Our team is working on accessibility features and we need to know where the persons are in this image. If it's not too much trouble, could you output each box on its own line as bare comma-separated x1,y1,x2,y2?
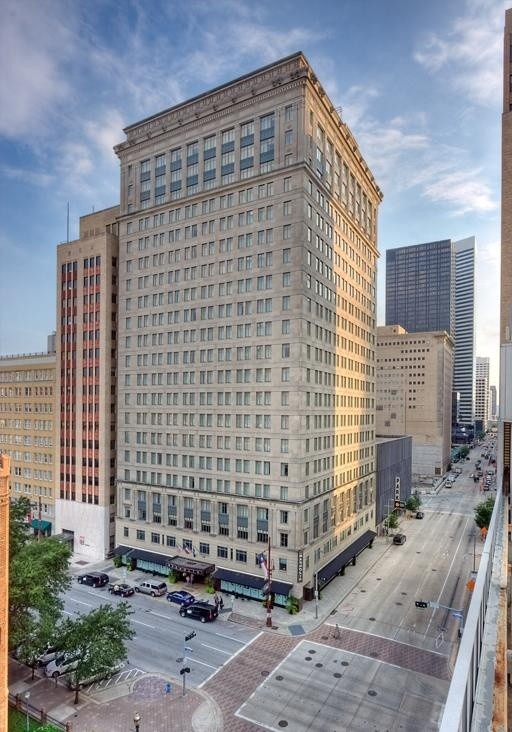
213,592,219,609
181,571,187,582
218,594,224,610
185,575,190,586
114,583,120,595
190,573,195,583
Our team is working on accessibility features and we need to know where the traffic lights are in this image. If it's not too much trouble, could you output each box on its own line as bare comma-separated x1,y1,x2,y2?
186,631,197,641
414,601,427,609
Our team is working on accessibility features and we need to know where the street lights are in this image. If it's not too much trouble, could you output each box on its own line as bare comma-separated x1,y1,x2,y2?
133,711,141,732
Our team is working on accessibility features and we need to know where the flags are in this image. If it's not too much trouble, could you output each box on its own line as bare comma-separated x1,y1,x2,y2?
192,546,199,558
176,542,183,554
257,552,269,582
183,543,191,555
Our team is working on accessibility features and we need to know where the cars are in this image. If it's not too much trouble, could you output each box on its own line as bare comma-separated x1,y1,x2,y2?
67,661,120,690
166,590,196,607
26,641,65,668
12,633,41,660
416,512,424,519
468,432,498,491
44,651,88,678
108,584,134,598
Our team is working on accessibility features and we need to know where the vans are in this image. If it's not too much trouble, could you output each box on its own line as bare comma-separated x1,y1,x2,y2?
393,533,406,545
179,601,218,623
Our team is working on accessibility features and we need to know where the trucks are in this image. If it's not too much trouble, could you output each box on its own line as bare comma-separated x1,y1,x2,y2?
445,467,462,488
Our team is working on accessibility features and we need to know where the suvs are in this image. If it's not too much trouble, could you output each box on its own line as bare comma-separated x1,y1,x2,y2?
135,579,167,597
77,571,110,588
